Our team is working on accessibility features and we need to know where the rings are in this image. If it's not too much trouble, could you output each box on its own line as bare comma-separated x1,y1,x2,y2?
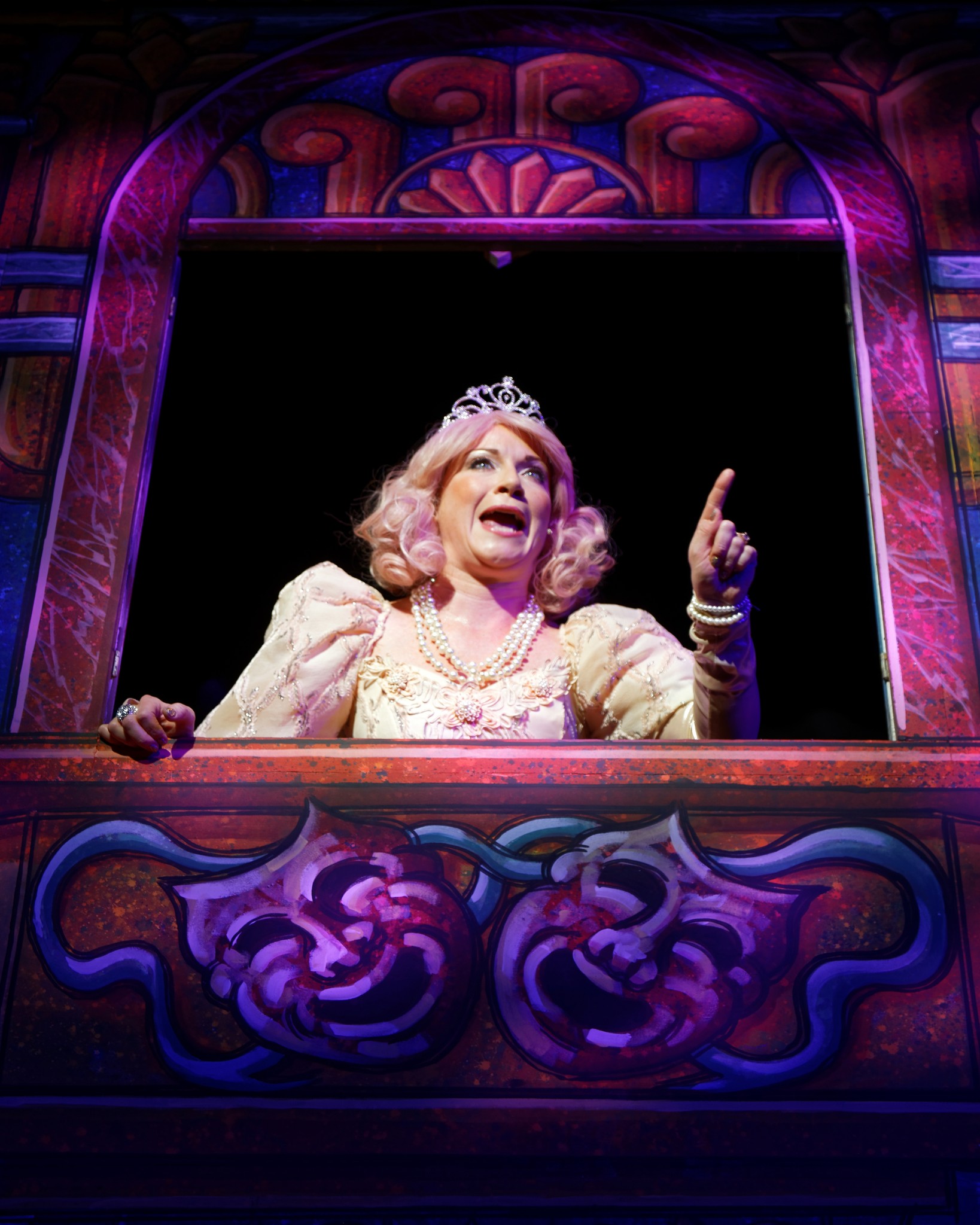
116,703,138,723
735,531,750,543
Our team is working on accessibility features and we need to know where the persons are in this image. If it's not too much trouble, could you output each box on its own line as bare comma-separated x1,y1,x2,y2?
95,373,760,759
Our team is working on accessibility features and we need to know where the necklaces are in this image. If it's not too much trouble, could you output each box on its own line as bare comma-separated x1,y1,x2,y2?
411,576,544,723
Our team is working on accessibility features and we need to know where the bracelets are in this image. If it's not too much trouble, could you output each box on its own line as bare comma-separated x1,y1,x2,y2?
684,591,752,643
